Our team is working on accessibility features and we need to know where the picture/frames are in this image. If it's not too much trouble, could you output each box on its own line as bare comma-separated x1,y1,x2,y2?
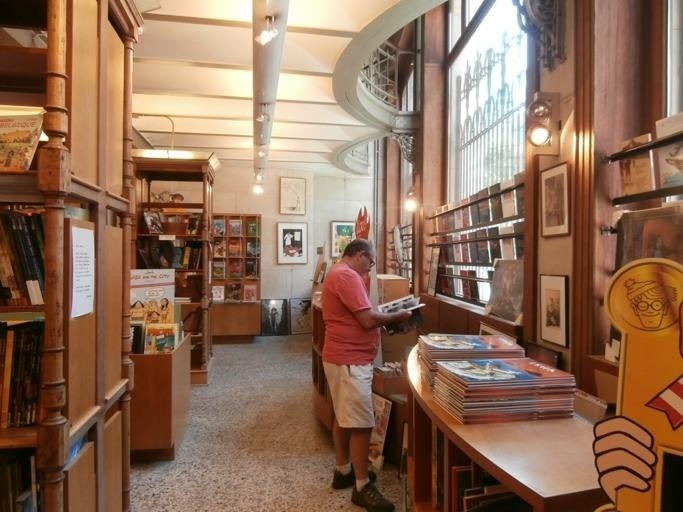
478,321,519,344
524,339,562,370
260,176,357,336
540,274,569,348
427,246,442,297
540,160,570,238
609,205,683,361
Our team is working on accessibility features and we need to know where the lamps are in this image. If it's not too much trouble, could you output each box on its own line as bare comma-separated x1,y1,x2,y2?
525,91,560,155
403,186,419,212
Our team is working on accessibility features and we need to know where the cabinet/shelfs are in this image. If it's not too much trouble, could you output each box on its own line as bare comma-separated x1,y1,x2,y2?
128,332,191,461
0,1,145,511
419,297,512,335
162,212,261,344
131,148,221,387
311,291,417,445
407,342,611,512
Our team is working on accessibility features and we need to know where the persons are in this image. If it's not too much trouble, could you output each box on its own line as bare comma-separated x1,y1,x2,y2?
623,280,676,329
284,231,295,255
592,414,657,506
319,236,397,511
269,304,279,336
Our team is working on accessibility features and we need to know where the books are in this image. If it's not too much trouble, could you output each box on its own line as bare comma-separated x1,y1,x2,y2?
0,102,44,507
211,219,258,302
129,208,202,355
615,132,656,197
451,464,532,511
655,111,683,188
377,295,426,335
415,333,576,424
425,170,525,302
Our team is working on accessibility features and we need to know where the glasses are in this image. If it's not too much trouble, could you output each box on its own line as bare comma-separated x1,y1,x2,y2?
368,258,375,268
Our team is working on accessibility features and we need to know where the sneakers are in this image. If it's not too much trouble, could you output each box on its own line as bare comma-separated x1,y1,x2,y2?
332,463,376,489
351,479,395,512
